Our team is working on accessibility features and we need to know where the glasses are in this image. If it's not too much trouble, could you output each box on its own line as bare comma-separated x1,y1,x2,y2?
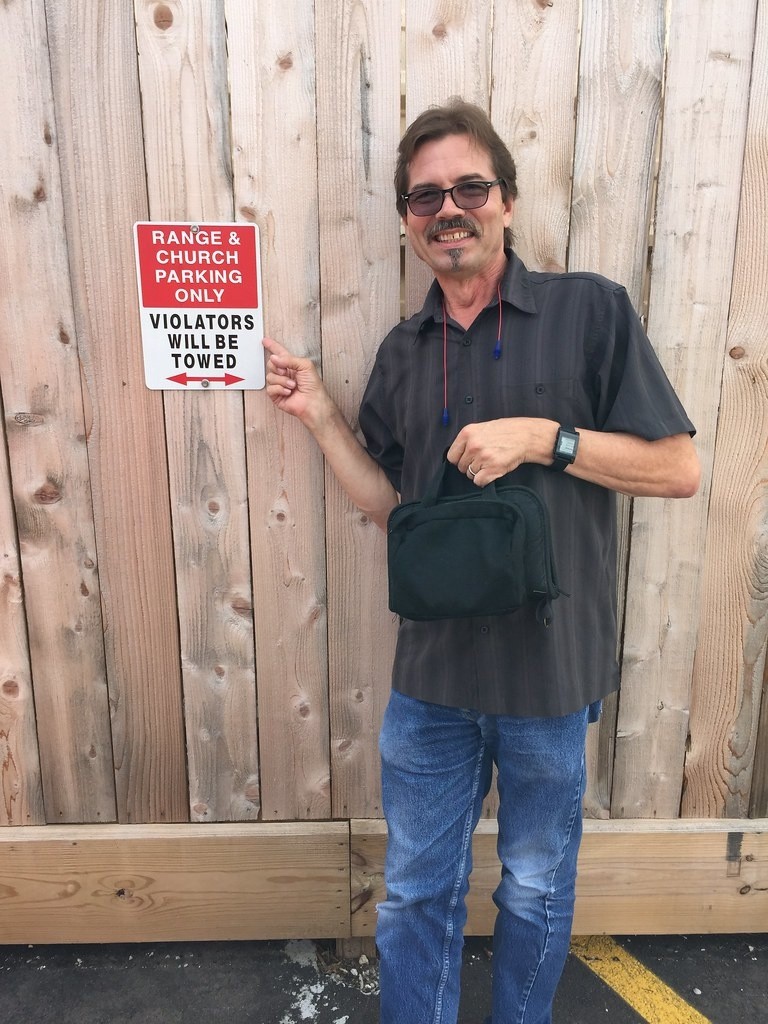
400,176,503,217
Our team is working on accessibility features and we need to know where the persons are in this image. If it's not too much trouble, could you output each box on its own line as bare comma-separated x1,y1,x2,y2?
256,92,703,1021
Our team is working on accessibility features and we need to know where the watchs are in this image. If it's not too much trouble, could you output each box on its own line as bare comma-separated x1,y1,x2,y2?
545,420,581,473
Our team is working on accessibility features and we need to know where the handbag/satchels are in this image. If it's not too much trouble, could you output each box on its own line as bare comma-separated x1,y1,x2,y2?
386,445,571,628
